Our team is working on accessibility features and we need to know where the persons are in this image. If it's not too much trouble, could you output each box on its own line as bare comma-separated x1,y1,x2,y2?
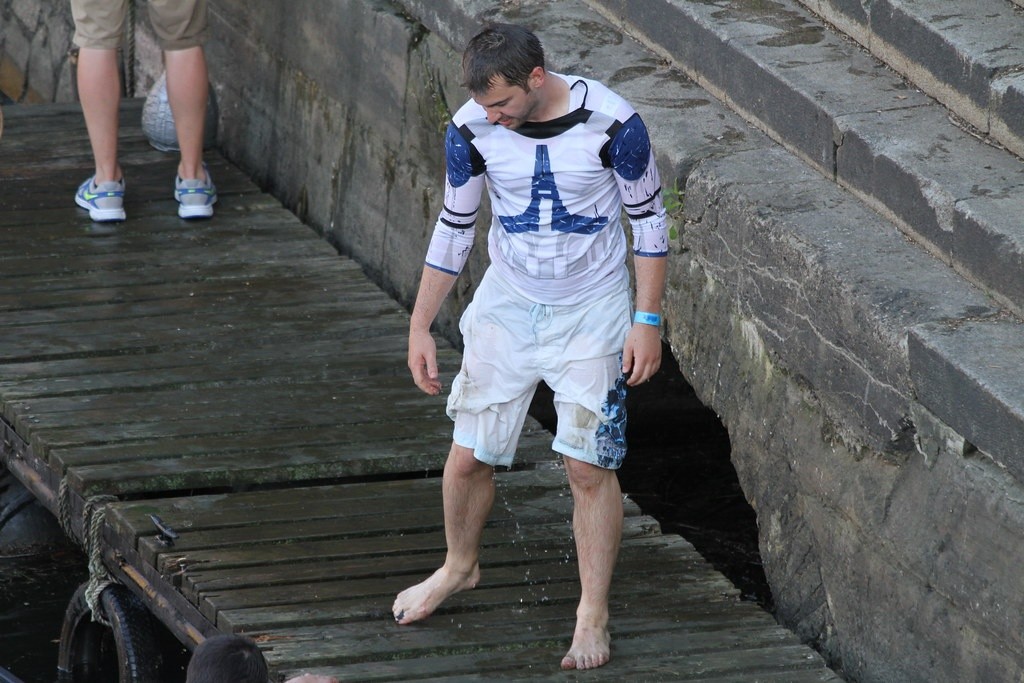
186,634,337,683
390,24,668,669
74,1,223,222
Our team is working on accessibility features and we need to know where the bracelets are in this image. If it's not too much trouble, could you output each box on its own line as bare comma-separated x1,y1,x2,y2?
634,311,660,327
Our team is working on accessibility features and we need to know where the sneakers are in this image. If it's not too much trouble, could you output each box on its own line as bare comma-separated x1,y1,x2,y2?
74,172,127,222
174,164,217,218
